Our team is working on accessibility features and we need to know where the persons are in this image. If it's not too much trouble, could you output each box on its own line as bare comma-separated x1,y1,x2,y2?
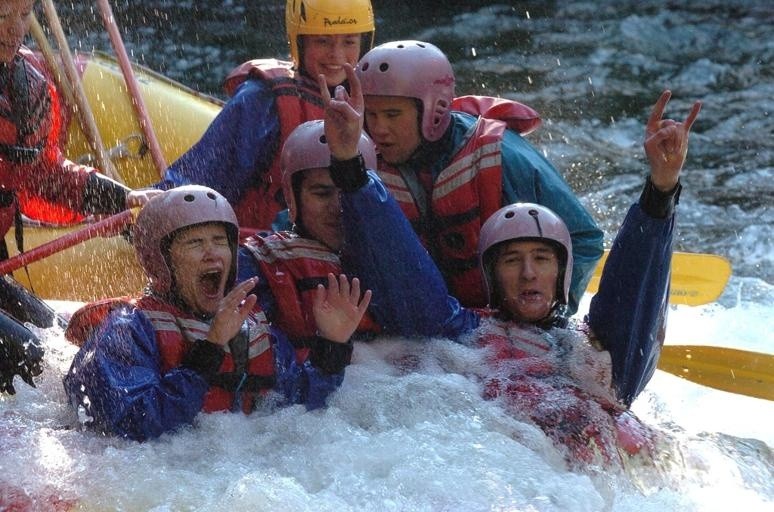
63,185,372,443
320,64,702,469
1,0,166,396
234,120,377,360
156,0,372,207
355,40,604,314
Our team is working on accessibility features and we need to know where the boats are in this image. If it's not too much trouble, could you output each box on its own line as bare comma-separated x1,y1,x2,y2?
0,49,773,306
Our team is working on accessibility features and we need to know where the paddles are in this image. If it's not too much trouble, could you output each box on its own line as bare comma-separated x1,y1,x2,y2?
656,344,773,402
586,248,730,306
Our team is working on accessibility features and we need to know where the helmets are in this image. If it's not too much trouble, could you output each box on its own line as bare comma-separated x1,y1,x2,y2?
475,201,572,311
285,0,375,68
279,118,378,231
349,39,455,146
129,183,240,299
